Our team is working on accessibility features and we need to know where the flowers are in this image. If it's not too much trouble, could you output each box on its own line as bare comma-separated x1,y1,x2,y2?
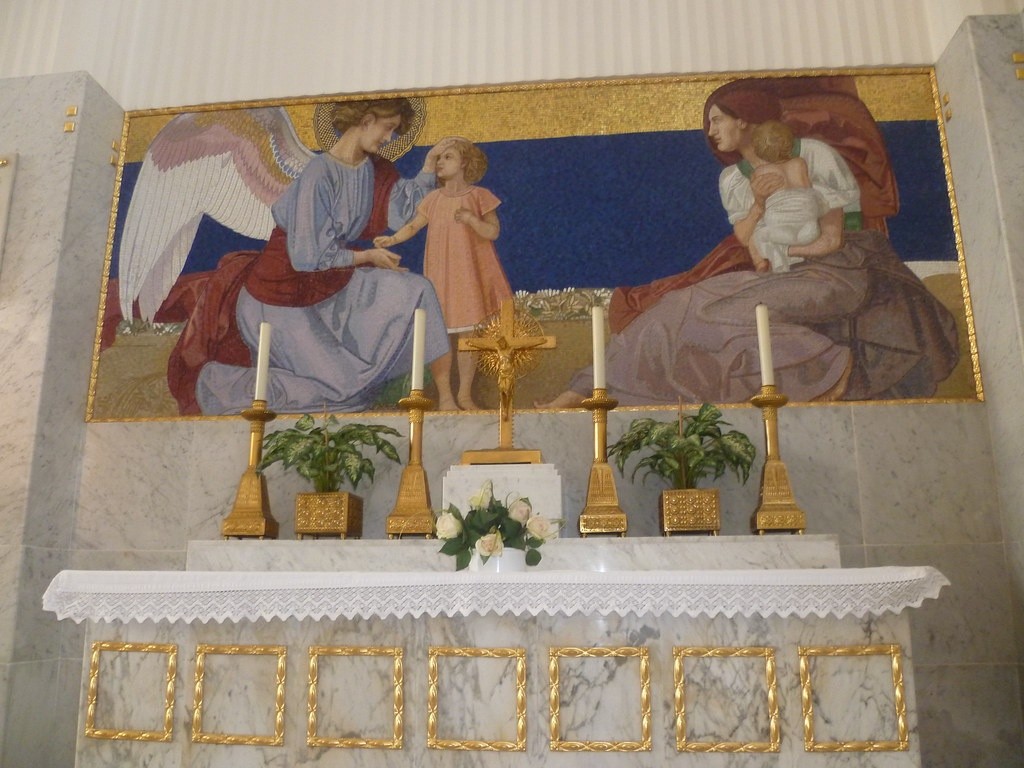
436,481,560,573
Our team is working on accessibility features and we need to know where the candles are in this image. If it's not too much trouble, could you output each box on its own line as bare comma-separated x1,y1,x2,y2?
755,302,774,390
407,305,428,389
590,305,606,393
254,322,273,403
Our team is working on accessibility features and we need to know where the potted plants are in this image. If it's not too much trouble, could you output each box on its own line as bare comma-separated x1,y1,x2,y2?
259,402,405,540
606,396,756,534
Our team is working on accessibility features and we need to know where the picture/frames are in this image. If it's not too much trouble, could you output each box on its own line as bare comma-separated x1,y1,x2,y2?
82,64,985,422
